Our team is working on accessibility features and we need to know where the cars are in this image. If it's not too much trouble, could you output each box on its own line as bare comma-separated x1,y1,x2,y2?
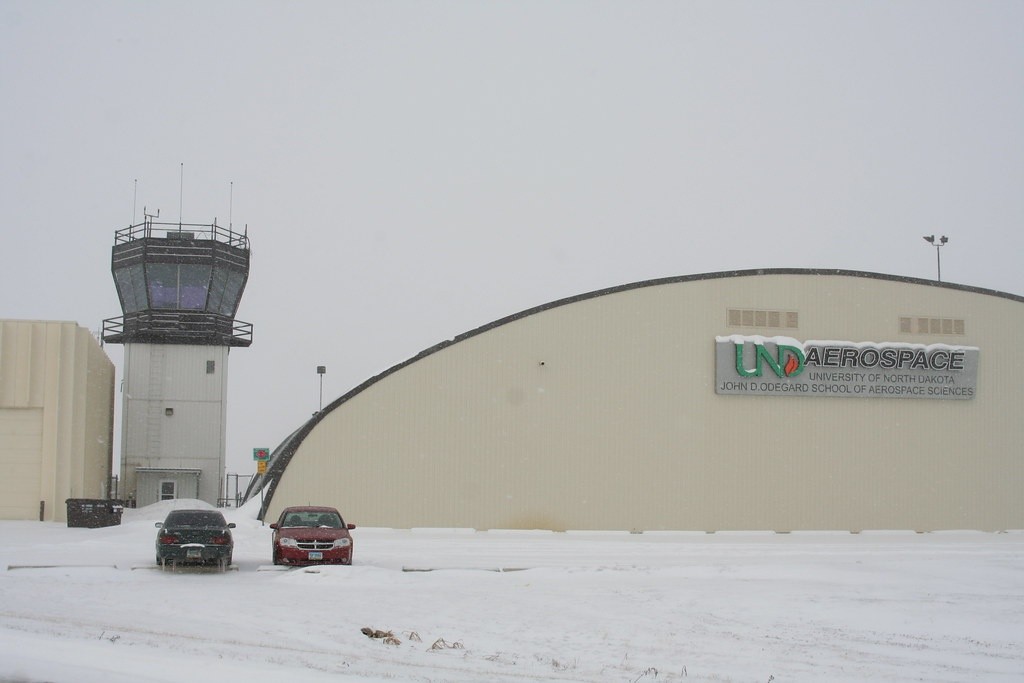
270,506,355,567
154,510,236,565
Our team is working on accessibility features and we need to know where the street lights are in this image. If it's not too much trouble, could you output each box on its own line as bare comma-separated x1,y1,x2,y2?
923,235,948,281
317,366,326,410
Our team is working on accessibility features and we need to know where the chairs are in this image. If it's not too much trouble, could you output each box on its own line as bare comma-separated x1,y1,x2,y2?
318,515,332,525
290,515,302,526
